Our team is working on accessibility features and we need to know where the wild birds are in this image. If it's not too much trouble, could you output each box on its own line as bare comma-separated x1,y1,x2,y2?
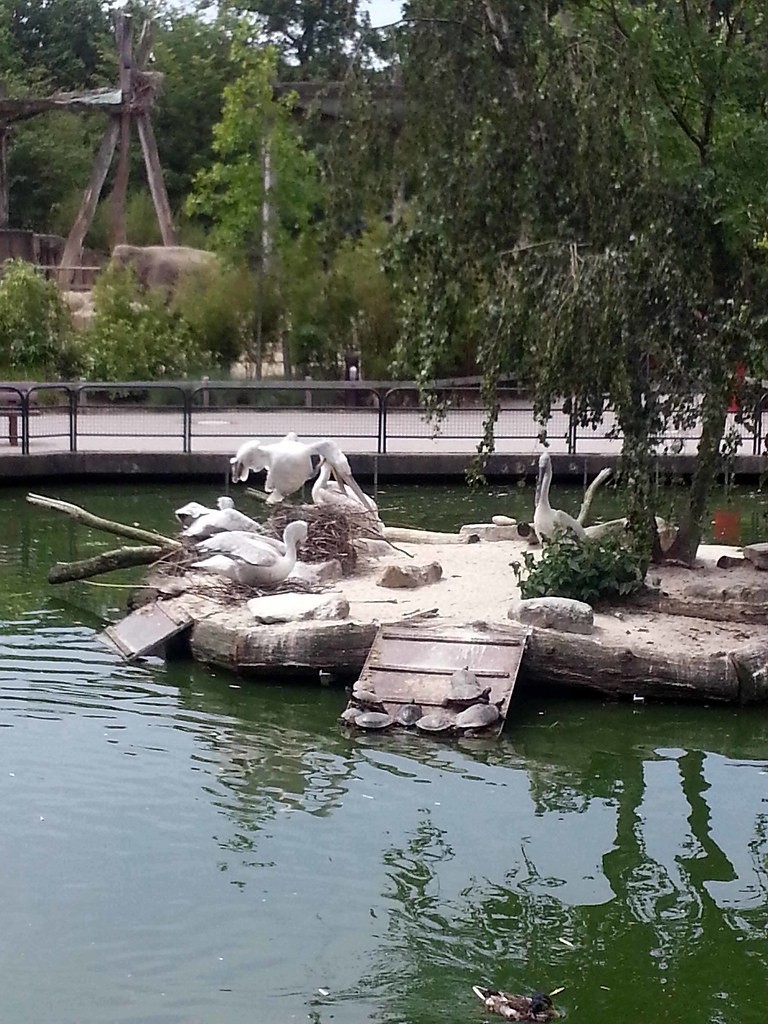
530,451,612,545
229,431,379,521
174,496,268,537
190,520,309,595
